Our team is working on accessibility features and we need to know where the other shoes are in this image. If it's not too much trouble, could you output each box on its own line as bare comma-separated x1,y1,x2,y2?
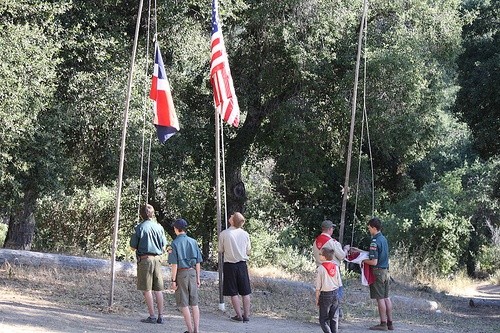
388,324,394,330
230,314,243,322
156,316,164,323
370,324,388,330
242,314,249,323
141,316,157,323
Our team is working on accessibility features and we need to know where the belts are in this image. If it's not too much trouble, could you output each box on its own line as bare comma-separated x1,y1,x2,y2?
177,267,194,271
139,254,159,259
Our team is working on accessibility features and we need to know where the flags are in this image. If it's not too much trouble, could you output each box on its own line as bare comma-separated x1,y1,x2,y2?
343,247,374,286
209,0,240,128
149,42,181,142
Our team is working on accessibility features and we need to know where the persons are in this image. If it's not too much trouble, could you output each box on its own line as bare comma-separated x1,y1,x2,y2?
129,203,167,324
314,247,344,333
312,219,351,318
217,212,252,322
360,219,394,330
167,218,203,333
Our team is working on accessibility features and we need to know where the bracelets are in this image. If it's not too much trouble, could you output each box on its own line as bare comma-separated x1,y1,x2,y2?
171,279,176,282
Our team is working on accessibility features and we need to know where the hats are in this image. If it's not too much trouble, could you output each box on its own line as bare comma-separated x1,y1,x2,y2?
321,221,336,229
170,218,188,230
